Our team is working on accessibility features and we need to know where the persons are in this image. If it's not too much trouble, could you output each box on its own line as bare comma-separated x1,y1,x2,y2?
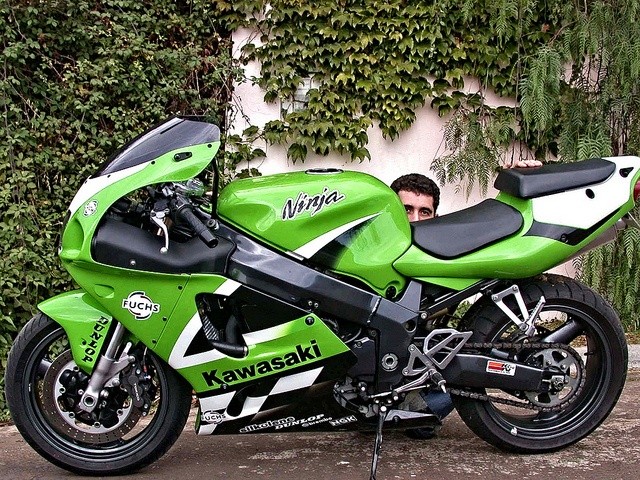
356,173,454,439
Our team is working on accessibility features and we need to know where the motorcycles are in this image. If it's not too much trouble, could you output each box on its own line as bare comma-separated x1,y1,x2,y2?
4,117,640,476
